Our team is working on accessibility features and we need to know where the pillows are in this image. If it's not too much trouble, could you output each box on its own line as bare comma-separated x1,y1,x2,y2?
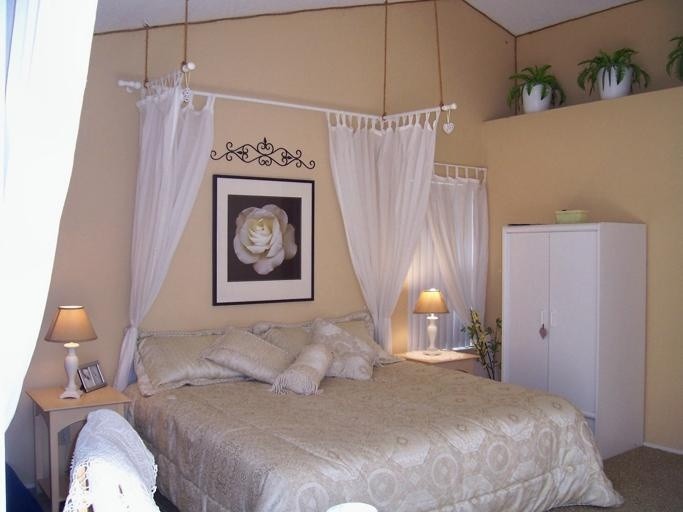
137,313,405,397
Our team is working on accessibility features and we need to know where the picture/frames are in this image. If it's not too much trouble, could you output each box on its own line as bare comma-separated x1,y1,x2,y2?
78,363,107,394
212,174,315,306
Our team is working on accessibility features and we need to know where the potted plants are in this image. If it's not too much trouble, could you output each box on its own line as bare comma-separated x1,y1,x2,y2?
504,47,652,115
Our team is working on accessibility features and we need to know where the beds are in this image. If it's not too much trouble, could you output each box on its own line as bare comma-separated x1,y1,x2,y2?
130,309,581,511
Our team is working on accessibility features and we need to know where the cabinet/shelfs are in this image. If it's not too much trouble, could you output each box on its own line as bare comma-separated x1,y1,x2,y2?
502,227,647,460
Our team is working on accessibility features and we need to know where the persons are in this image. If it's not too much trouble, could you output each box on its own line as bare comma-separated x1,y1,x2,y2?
82,368,100,389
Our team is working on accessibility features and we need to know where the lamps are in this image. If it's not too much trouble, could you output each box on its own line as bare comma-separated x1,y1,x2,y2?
412,288,451,356
44,305,98,399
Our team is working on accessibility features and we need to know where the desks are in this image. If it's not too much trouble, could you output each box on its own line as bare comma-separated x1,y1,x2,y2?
26,383,132,512
406,350,479,375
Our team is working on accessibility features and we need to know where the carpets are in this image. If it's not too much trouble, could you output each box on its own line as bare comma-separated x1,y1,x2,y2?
550,444,681,511
